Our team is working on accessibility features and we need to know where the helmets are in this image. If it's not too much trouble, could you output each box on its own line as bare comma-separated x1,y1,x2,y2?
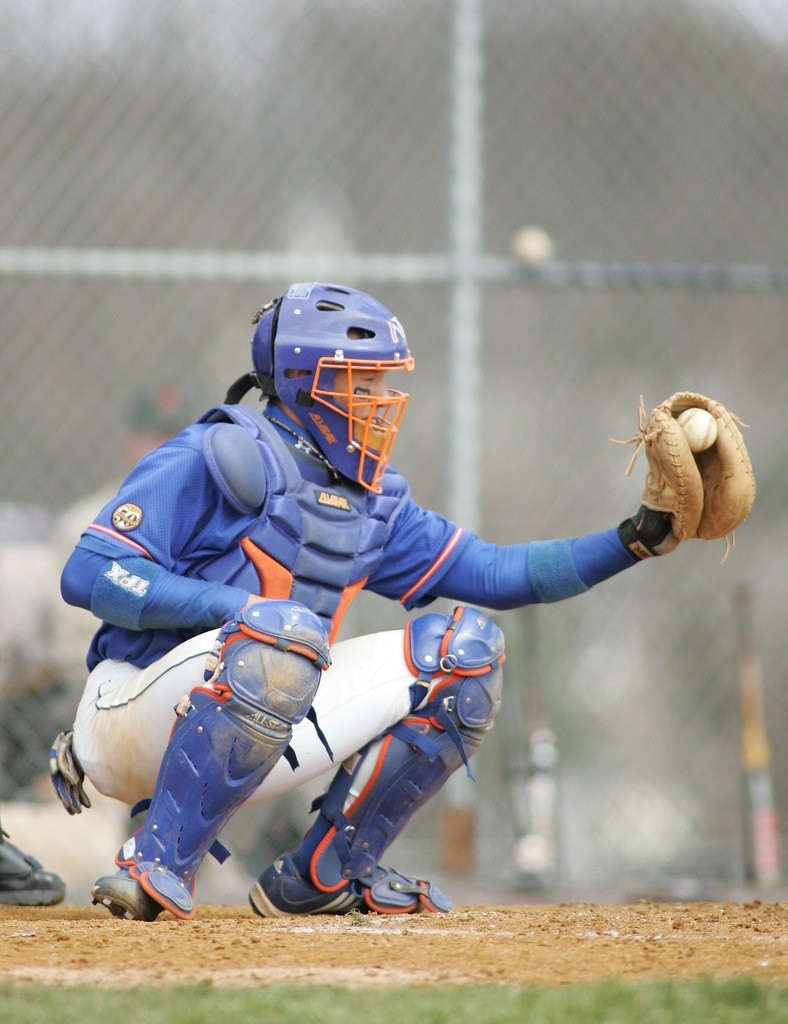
250,282,415,495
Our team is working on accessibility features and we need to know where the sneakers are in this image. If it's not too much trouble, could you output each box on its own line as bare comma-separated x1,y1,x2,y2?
248,846,424,917
90,866,160,922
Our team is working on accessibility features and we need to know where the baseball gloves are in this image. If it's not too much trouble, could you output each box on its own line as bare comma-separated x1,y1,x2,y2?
629,391,758,566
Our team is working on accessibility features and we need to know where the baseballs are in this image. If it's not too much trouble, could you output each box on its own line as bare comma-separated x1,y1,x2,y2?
674,406,721,453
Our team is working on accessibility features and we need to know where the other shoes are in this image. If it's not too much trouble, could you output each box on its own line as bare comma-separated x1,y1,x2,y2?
0,828,66,906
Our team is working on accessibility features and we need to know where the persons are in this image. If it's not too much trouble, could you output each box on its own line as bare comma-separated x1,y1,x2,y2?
56,286,757,925
